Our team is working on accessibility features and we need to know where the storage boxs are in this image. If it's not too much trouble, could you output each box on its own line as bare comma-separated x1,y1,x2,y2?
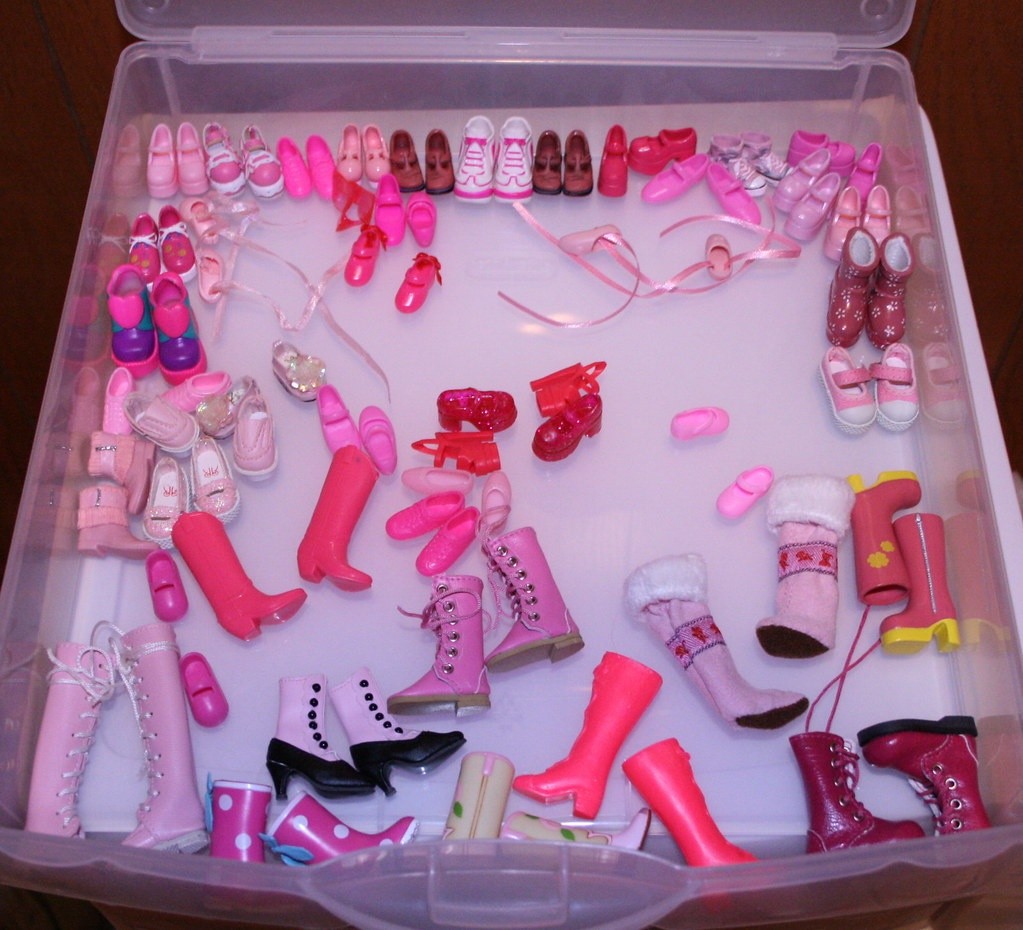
0,0,1023,930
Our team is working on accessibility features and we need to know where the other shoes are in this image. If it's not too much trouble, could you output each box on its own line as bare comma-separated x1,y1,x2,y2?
44,115,994,871
